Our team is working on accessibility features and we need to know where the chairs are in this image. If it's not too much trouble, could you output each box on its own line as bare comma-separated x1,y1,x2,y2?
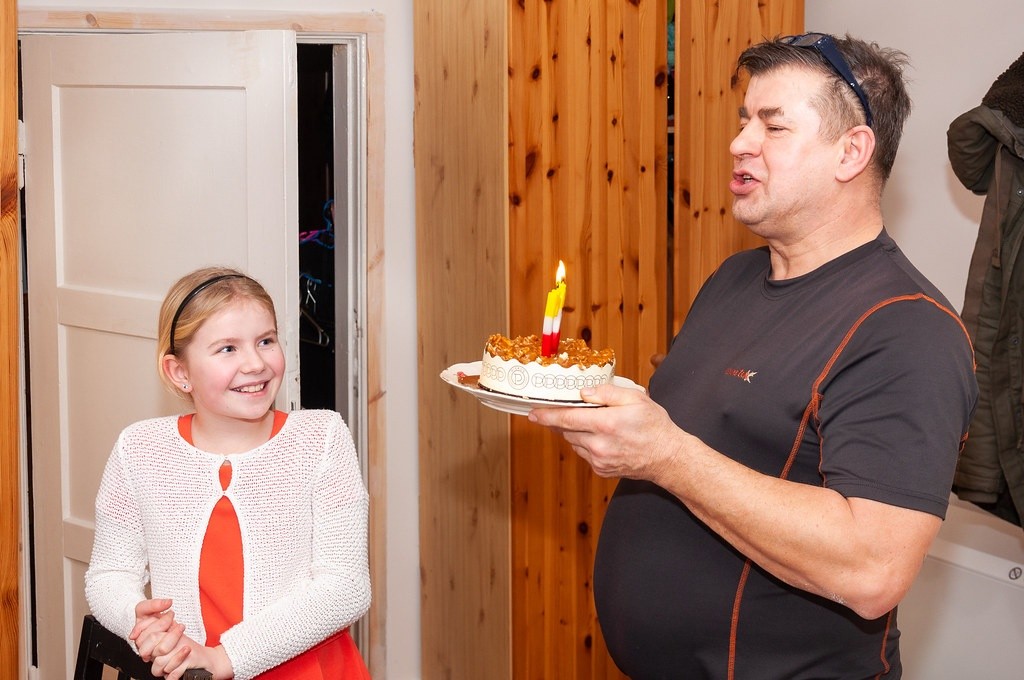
73,615,213,680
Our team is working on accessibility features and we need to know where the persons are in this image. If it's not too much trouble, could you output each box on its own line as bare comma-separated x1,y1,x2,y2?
85,266,375,680
526,38,976,680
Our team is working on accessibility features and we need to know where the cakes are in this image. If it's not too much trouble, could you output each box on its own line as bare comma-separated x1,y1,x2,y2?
477,333,617,400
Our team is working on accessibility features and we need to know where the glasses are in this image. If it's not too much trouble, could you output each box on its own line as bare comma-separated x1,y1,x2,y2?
775,35,872,125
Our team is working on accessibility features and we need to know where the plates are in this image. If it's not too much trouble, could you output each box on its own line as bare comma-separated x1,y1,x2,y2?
439,361,646,417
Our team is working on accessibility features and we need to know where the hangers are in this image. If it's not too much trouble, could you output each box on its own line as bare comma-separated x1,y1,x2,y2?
298,198,334,347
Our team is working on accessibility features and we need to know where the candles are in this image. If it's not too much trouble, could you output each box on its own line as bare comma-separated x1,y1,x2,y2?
542,260,568,356
